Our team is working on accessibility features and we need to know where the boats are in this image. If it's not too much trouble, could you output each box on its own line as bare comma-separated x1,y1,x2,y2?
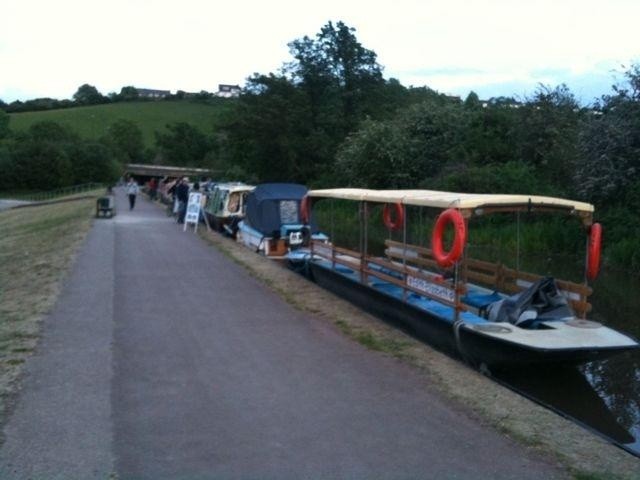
283,186,639,375
204,182,257,233
236,182,331,262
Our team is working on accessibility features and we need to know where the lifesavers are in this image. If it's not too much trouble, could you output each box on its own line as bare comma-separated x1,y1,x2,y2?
588,223,601,278
431,210,466,268
302,197,311,224
384,202,403,230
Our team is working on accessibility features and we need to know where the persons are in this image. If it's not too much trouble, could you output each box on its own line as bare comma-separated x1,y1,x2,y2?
126,177,139,210
149,176,217,224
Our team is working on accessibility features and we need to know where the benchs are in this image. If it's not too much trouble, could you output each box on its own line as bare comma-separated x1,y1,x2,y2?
309,238,594,330
